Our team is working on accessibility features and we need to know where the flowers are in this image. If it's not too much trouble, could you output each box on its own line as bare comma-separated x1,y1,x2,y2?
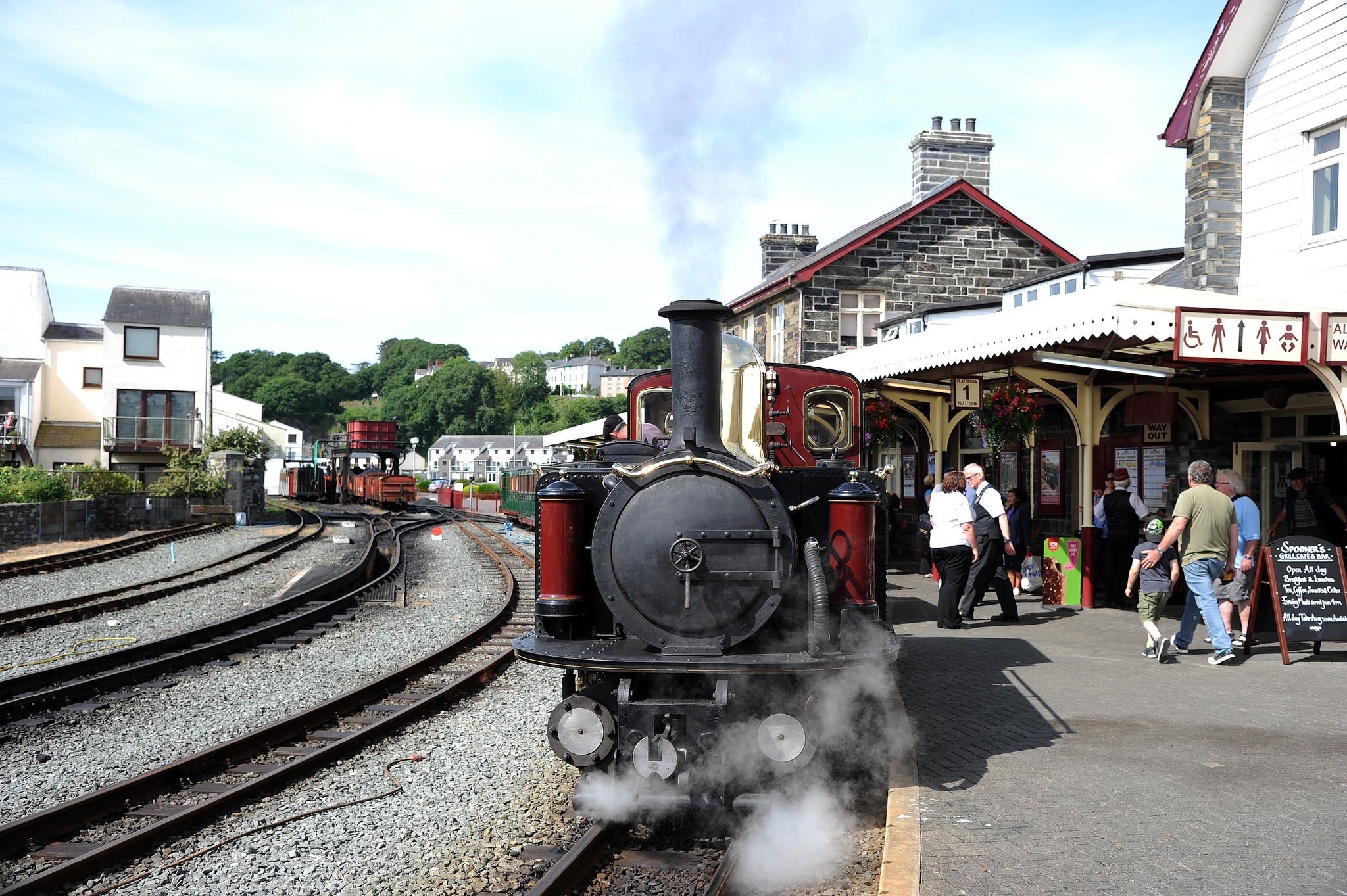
863,395,902,454
965,382,1044,449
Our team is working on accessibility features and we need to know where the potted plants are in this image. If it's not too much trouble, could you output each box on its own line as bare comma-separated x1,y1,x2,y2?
417,480,432,493
463,485,479,497
477,483,501,500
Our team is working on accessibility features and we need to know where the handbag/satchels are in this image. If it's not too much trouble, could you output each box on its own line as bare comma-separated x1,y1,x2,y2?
1021,550,1043,591
1092,526,1103,553
917,513,933,540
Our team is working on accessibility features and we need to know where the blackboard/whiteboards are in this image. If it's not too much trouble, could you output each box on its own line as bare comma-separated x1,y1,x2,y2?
1245,534,1347,640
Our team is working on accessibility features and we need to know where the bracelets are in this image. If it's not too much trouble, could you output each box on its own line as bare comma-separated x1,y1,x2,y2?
1097,496,1101,499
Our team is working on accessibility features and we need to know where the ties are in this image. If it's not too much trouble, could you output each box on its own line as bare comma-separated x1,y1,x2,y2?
1144,515,1165,543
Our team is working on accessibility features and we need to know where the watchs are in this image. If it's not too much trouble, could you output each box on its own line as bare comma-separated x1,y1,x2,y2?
1004,538,1012,543
1156,545,1167,554
1242,554,1253,560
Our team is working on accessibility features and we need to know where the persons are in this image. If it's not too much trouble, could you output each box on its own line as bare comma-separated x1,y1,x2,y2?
1092,468,1165,609
1125,515,1180,663
1002,489,1033,595
885,502,897,540
1266,467,1347,544
603,415,670,448
1204,469,1262,647
1140,459,1239,664
958,464,1020,624
929,471,972,629
0,411,18,443
920,467,1010,607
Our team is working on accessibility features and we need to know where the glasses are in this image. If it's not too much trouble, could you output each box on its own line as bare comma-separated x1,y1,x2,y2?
1105,476,1114,482
1215,481,1229,485
966,472,979,480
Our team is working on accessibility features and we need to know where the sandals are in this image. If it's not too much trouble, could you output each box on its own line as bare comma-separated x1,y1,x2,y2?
1232,634,1257,646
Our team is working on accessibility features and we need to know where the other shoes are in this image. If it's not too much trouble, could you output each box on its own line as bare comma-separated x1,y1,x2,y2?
1013,587,1022,596
991,614,1019,623
961,615,974,620
943,623,971,630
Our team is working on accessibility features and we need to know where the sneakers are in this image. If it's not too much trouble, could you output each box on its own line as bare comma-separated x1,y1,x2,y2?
1155,636,1170,663
1170,634,1189,654
1207,648,1235,665
1142,644,1157,658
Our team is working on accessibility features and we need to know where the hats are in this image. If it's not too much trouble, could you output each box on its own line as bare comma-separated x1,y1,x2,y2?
1114,468,1130,481
603,414,623,441
1287,467,1308,480
943,466,957,475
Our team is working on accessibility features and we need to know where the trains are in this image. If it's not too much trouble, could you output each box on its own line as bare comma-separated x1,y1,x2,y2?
278,467,325,501
497,299,894,831
322,472,416,510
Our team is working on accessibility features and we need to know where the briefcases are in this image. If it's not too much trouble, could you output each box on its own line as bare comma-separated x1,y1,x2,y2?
1204,633,1235,643
